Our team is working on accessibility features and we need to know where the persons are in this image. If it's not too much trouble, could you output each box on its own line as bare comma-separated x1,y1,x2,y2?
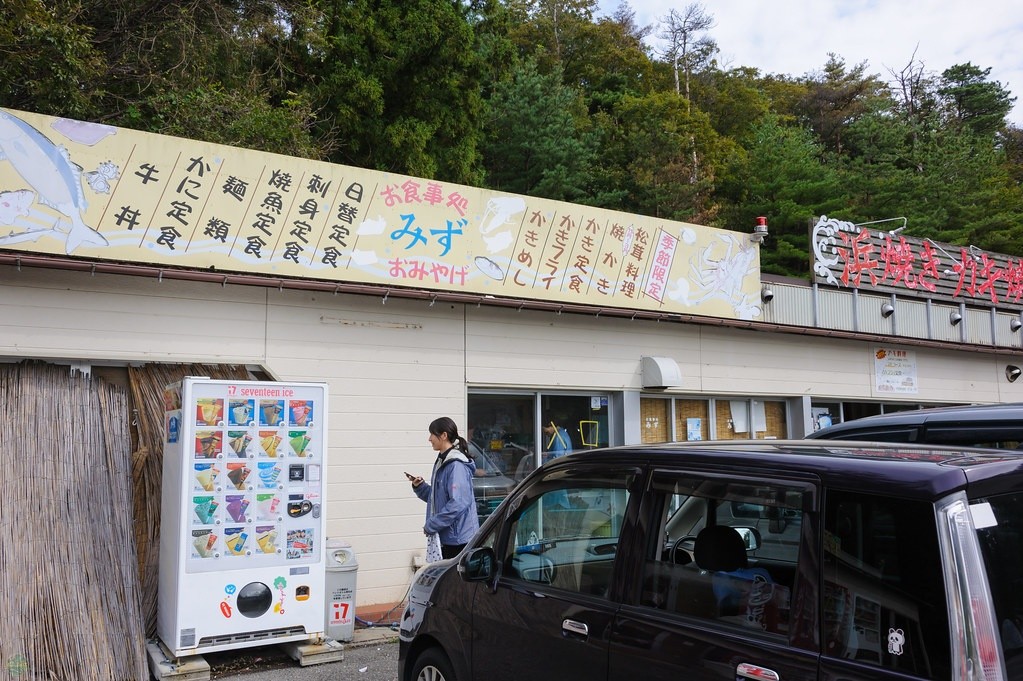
542,417,572,538
406,417,479,559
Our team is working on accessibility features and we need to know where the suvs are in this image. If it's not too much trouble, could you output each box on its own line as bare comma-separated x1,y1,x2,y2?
514,450,581,484
469,441,516,528
398,405,1023,681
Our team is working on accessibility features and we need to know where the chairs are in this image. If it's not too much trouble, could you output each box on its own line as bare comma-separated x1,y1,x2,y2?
694,526,778,631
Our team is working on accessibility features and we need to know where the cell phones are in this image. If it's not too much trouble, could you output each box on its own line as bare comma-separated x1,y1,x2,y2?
404,472,422,483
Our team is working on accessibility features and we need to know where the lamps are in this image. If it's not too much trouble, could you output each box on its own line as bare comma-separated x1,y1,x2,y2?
950,312,962,326
761,288,773,304
881,304,894,318
1011,319,1022,332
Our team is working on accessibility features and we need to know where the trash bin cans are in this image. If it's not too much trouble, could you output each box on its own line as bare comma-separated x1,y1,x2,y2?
590,520,612,537
325,542,359,644
